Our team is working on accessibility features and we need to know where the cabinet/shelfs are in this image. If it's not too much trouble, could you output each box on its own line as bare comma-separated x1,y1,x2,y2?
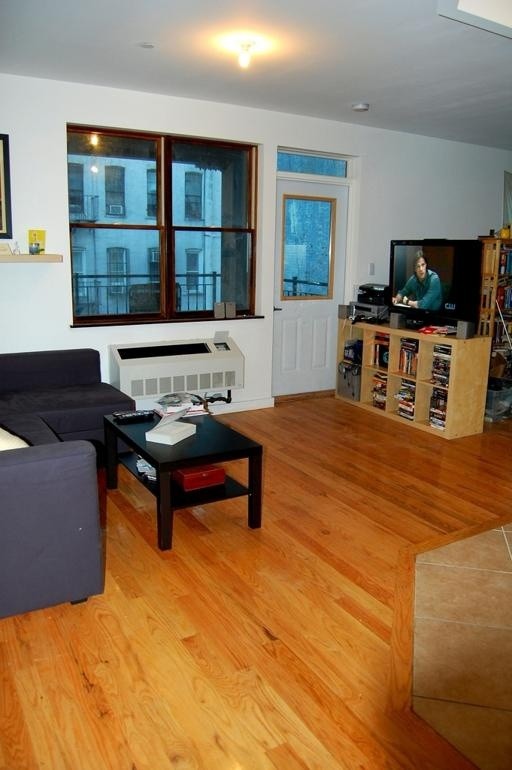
335,318,492,440
477,236,512,351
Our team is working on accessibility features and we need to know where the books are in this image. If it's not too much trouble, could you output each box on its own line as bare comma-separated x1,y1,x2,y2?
392,380,416,421
428,388,447,431
482,289,511,309
145,408,197,445
482,320,512,344
164,404,204,412
397,339,417,376
154,407,209,417
431,345,451,387
373,332,390,370
370,372,387,410
500,253,510,274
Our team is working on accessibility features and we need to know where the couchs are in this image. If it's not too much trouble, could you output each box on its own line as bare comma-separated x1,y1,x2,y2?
0,348,136,618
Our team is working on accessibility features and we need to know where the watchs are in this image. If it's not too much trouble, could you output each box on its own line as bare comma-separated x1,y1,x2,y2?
406,299,409,305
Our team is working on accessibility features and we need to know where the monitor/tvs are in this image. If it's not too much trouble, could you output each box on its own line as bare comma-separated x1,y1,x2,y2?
391,238,484,326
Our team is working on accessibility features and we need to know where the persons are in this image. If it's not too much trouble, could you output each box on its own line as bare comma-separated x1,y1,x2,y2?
392,249,443,311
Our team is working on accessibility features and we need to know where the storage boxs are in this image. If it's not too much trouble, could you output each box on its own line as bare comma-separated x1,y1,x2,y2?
173,467,225,492
485,384,512,422
337,371,360,401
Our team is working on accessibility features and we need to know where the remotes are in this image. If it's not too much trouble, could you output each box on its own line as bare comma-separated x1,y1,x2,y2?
118,410,155,419
112,410,137,417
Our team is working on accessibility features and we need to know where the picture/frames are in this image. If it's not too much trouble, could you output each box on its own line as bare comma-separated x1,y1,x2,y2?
1,133,13,239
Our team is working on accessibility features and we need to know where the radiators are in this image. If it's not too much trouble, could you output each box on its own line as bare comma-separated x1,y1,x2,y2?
108,332,245,400
